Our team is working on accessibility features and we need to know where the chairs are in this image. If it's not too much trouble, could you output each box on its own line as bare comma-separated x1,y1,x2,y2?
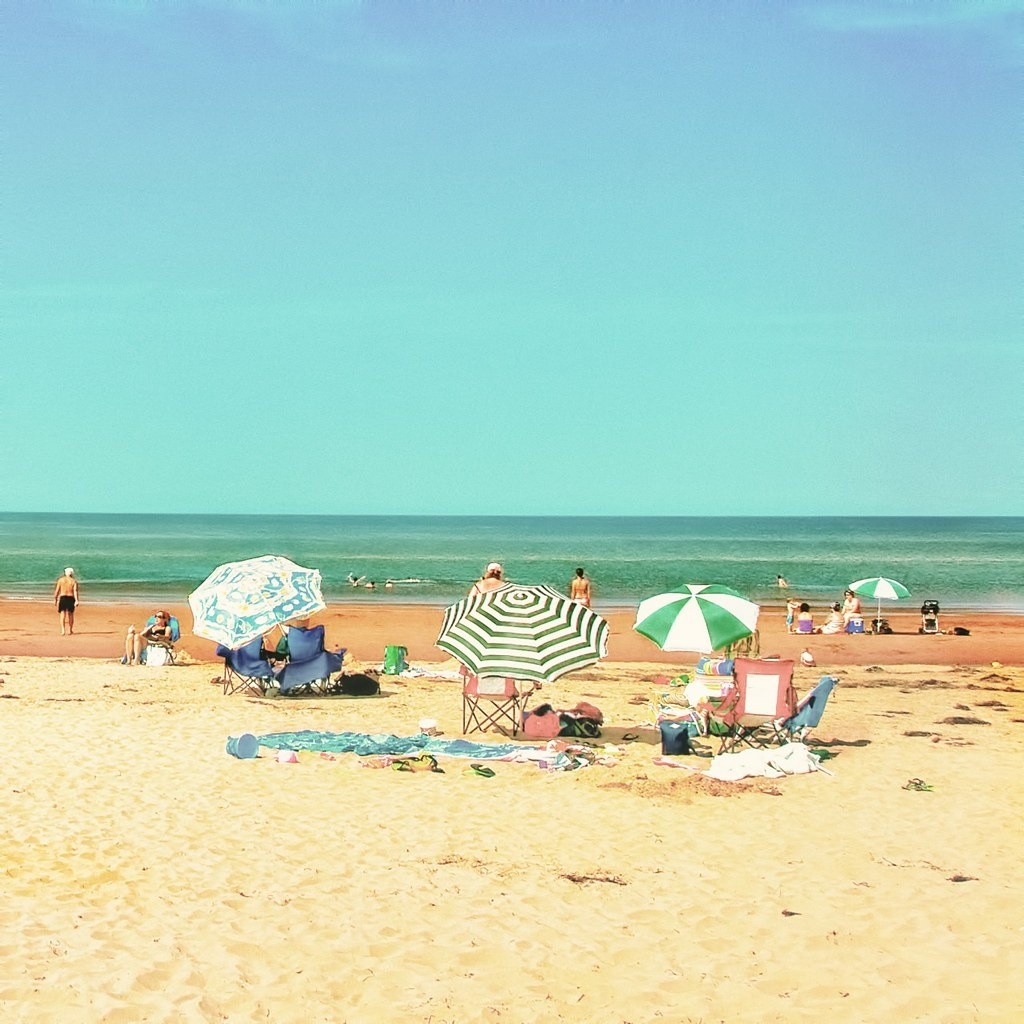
719,676,834,754
460,664,542,740
121,615,180,664
277,625,329,695
723,657,796,756
217,637,275,698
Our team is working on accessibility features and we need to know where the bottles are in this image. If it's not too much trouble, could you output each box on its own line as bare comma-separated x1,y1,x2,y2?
334,644,339,652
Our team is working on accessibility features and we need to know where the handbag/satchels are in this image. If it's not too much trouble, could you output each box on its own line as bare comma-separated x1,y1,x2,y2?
954,627,970,635
384,644,410,674
557,713,602,737
651,705,708,737
146,643,170,666
847,619,864,633
520,704,560,739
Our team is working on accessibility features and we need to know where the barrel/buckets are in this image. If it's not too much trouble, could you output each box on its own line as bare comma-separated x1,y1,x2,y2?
419,718,437,737
226,733,259,758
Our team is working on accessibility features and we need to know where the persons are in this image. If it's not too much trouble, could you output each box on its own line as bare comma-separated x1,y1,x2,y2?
364,581,376,587
466,562,507,599
346,574,367,587
777,574,786,590
54,567,81,638
841,588,863,628
274,618,310,661
568,568,592,609
124,610,173,665
384,580,392,588
796,603,815,635
784,597,801,633
813,602,847,634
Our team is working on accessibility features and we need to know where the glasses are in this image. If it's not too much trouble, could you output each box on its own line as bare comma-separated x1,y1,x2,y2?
155,614,164,619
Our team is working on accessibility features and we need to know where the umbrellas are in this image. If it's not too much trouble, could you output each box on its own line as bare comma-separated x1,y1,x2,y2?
632,583,761,659
847,576,912,635
434,581,612,734
186,553,328,652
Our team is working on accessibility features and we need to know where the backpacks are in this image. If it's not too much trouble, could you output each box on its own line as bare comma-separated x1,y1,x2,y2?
659,719,704,756
391,751,443,774
327,674,381,697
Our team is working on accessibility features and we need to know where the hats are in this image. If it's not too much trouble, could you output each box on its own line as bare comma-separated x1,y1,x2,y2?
488,563,503,575
